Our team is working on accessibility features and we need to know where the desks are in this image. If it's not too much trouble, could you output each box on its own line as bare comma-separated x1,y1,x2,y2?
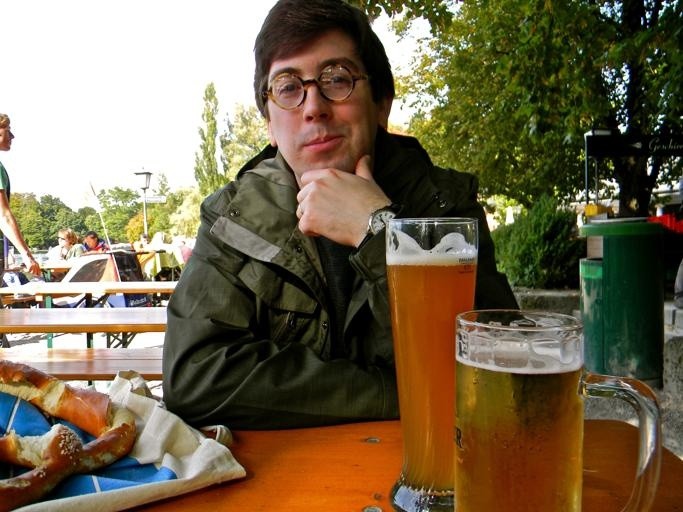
0,280,179,347
0,307,168,333
0,345,164,383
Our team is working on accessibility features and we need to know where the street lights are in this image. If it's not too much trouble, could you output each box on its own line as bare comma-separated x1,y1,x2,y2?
134,166,153,235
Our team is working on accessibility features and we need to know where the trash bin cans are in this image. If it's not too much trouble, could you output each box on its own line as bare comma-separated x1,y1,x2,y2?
576,218,666,394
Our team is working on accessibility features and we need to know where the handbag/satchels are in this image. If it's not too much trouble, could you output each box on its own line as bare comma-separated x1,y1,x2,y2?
1,370,248,512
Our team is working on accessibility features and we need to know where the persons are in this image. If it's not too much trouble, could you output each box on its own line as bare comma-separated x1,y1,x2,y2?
82,231,107,255
48,228,86,261
161,0,522,431
0,112,41,288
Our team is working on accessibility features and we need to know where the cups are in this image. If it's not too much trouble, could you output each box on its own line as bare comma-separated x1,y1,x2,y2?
455,308,663,508
386,217,478,507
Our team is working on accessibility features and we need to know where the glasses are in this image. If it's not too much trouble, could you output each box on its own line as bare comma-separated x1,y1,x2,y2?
57,237,66,241
262,64,370,110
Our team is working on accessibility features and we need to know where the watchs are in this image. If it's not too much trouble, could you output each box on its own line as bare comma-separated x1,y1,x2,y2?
356,202,403,248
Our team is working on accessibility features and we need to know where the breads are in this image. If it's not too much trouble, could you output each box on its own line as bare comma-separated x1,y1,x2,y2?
0,423,83,510
0,359,136,472
